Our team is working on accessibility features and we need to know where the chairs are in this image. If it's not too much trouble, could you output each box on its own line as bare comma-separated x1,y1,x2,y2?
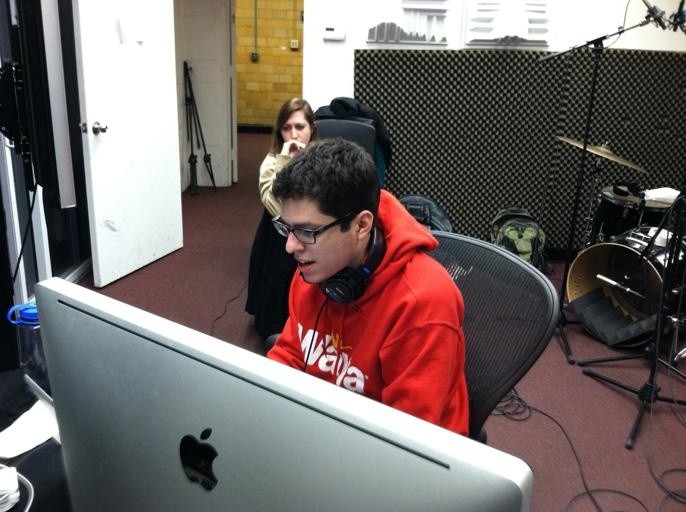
413,221,564,449
309,116,380,172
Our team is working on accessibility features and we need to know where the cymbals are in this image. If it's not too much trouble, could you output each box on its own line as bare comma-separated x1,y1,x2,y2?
556,135,649,175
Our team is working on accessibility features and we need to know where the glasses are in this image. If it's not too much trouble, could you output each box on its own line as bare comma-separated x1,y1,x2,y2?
272,213,359,245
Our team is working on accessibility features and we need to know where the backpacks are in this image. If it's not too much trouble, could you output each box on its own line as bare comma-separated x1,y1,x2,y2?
491,207,547,273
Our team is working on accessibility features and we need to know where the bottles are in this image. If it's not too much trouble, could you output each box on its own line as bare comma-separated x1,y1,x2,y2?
4,303,51,398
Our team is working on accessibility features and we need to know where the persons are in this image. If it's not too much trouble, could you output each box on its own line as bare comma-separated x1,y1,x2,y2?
266,137,469,436
243,98,317,337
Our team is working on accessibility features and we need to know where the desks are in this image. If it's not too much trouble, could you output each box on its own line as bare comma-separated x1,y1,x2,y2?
0,360,77,512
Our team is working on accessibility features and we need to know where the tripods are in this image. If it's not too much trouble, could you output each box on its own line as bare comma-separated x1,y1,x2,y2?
537,18,653,365
581,188,686,449
182,62,218,196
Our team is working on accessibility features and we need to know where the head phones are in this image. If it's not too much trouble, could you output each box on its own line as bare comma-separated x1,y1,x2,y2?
319,217,387,304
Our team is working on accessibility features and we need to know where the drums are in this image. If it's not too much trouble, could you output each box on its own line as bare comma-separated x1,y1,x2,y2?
565,187,686,348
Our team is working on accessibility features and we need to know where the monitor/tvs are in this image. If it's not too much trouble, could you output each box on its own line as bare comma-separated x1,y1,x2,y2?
34,276,535,512
